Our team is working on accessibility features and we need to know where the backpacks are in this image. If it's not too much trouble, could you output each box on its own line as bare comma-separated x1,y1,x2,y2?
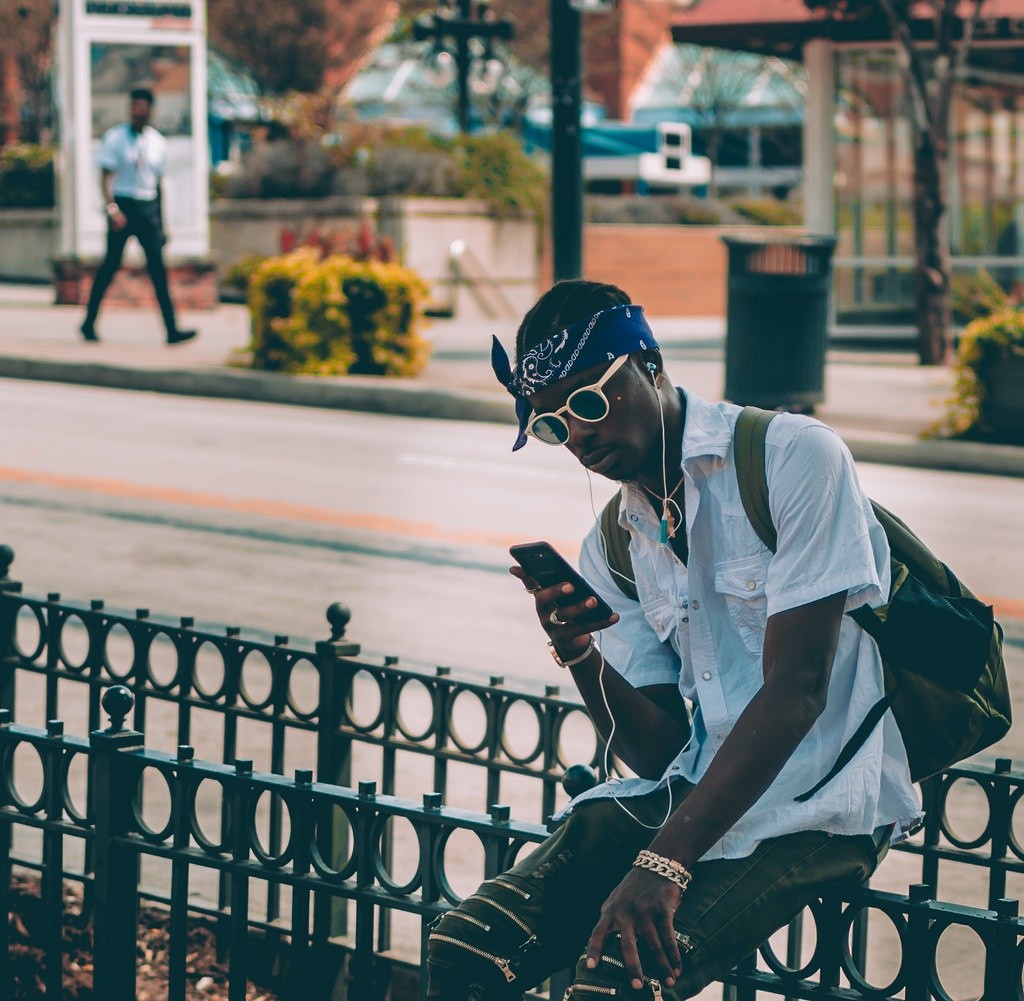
599,403,1012,783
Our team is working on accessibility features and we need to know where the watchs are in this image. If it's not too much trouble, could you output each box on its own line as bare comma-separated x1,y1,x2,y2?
547,636,596,668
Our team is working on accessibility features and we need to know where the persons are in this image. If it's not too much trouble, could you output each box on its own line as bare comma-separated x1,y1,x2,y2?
424,282,925,1001
80,88,197,345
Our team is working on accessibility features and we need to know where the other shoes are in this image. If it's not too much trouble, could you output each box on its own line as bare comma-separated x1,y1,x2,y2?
82,321,96,340
168,330,195,343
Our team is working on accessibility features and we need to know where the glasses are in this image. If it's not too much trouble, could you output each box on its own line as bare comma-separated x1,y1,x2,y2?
523,354,628,446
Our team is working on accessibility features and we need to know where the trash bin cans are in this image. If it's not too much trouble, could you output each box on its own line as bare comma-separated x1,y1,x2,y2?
719,233,837,417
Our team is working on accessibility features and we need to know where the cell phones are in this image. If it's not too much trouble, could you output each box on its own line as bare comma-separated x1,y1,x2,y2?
509,541,613,622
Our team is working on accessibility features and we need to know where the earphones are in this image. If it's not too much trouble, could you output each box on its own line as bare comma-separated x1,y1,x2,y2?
646,362,656,374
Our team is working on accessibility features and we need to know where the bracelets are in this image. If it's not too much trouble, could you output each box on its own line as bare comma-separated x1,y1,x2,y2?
633,850,692,892
